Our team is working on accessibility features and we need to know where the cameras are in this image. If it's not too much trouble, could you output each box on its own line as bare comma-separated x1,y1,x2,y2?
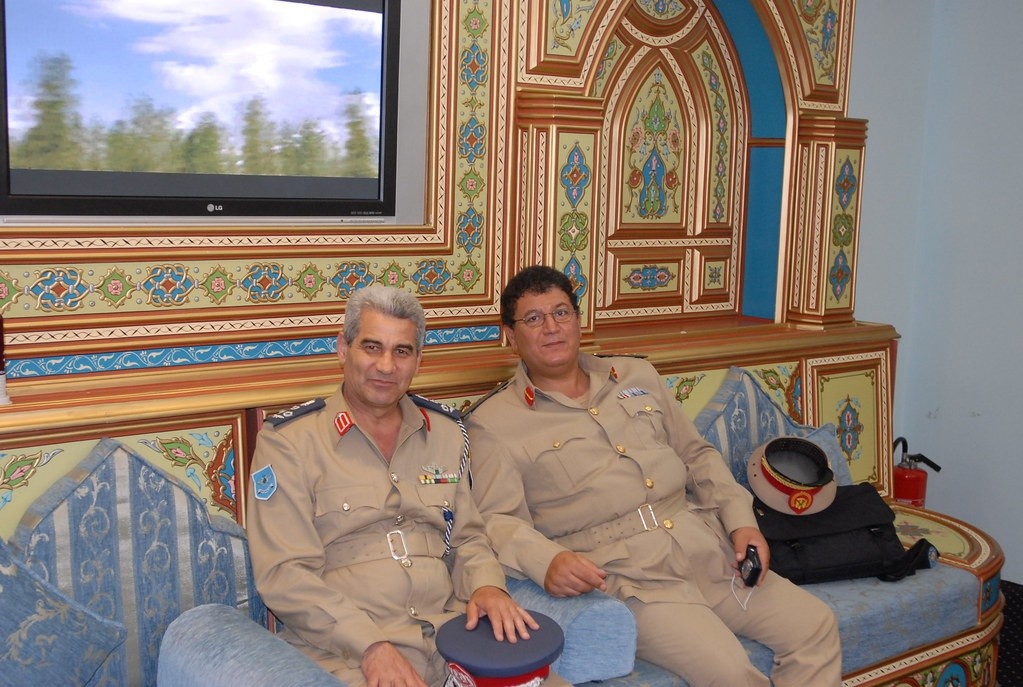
738,547,761,587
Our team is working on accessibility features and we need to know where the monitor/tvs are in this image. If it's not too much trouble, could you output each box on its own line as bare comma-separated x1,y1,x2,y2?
1,1,402,216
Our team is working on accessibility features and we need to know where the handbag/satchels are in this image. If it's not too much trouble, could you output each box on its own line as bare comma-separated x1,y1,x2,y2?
751,483,939,584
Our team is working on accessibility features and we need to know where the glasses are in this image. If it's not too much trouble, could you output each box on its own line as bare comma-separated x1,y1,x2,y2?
510,309,579,327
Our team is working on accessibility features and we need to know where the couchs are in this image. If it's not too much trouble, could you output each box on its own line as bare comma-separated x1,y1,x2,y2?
0,365,1008,687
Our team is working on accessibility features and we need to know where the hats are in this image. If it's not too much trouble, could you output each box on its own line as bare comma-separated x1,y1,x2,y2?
747,437,838,515
436,608,565,687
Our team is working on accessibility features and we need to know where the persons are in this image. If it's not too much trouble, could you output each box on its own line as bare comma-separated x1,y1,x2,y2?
457,266,845,686
249,284,579,687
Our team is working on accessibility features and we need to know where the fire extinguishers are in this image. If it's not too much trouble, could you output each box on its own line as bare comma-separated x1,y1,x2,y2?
892,436,941,509
